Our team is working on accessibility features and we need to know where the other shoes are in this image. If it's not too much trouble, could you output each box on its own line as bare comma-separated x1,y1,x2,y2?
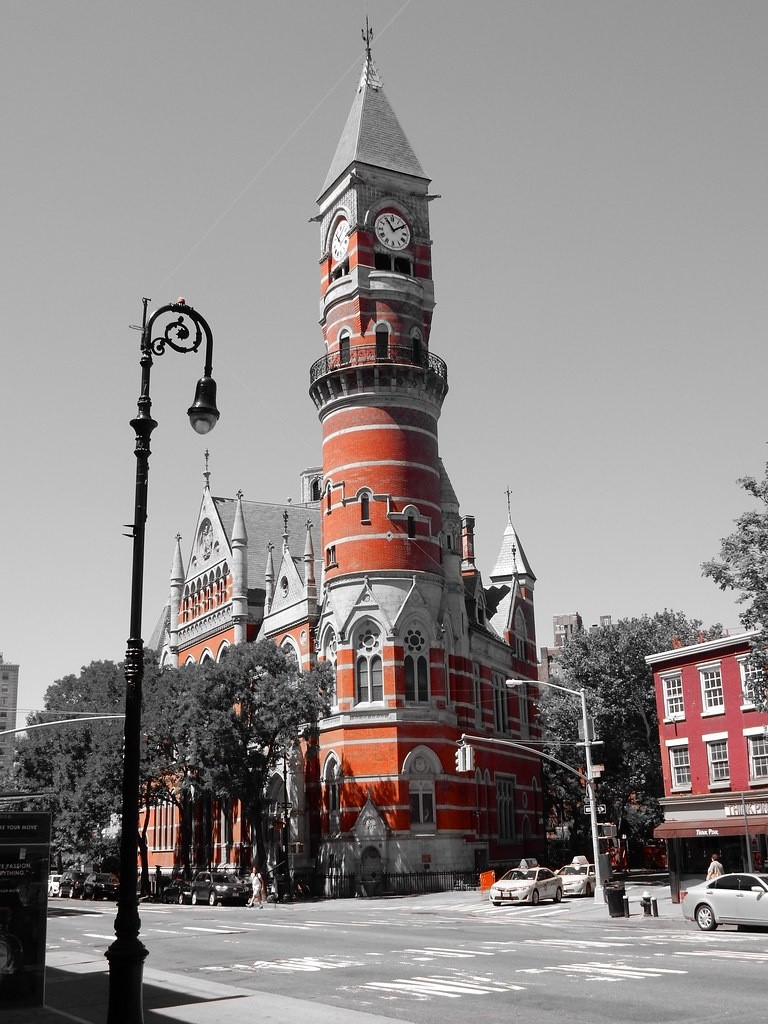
258,905,263,909
246,903,251,908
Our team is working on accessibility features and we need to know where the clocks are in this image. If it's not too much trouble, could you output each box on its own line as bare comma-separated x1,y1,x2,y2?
374,213,411,251
332,219,350,262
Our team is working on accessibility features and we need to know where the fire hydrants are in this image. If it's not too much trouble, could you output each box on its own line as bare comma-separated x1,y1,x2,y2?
641,890,652,918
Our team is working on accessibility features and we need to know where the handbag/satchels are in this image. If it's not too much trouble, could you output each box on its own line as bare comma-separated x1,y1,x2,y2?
710,862,722,879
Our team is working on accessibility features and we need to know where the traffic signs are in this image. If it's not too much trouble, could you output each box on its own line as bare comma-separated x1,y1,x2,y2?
276,802,292,808
584,804,606,815
582,795,599,804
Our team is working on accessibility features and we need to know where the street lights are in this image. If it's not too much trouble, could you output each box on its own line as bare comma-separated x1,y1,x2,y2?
105,296,219,1024
506,679,606,904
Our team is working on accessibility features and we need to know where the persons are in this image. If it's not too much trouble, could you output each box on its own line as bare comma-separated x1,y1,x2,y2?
706,854,724,881
245,866,263,908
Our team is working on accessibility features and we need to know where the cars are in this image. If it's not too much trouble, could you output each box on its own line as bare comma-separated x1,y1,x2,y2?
489,859,564,906
58,871,90,899
48,875,63,897
681,873,768,932
190,872,250,907
83,872,121,901
556,855,596,897
163,879,194,904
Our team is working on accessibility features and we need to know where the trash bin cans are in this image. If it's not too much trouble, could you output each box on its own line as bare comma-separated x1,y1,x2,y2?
276,875,292,899
606,880,625,917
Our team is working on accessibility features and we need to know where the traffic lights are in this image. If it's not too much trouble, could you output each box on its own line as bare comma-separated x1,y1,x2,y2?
466,746,476,771
601,825,617,837
455,749,466,772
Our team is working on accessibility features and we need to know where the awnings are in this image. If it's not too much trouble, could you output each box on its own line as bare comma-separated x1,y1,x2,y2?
654,817,768,839
